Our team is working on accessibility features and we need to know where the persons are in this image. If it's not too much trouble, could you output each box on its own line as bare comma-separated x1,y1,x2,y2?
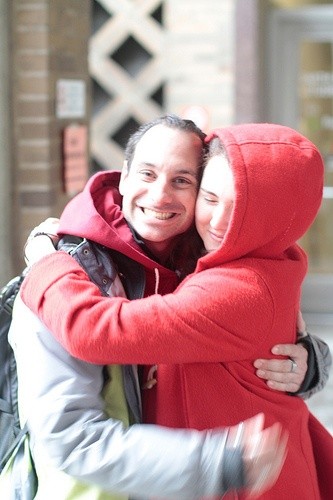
6,113,333,500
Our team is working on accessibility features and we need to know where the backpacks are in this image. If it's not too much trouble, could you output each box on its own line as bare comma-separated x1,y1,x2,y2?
0,233,95,500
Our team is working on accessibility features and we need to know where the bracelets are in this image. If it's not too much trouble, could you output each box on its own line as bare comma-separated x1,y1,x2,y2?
23,231,56,262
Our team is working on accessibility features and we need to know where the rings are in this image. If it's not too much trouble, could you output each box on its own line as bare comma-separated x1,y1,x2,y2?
288,358,295,373
